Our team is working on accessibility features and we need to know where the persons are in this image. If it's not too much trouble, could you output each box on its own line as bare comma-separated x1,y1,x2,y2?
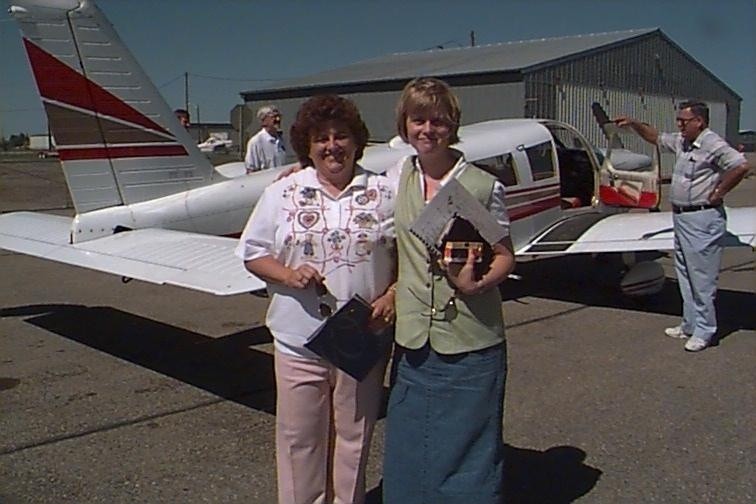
610,92,748,352
231,92,401,504
270,74,517,504
175,107,191,134
242,104,288,174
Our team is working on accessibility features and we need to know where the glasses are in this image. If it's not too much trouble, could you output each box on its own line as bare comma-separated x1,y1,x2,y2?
676,117,696,125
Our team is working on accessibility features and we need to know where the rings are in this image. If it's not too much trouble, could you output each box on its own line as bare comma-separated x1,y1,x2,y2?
384,317,390,323
389,321,394,325
299,276,304,282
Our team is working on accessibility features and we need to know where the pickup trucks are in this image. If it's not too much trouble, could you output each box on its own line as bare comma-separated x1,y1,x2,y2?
195,137,233,152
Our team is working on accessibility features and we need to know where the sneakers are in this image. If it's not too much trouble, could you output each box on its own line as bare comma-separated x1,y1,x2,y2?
664,325,690,340
684,336,711,352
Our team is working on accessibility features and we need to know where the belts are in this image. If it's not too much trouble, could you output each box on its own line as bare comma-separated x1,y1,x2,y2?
673,204,714,213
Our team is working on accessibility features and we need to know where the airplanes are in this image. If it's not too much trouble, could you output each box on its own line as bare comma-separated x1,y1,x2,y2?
0,0,756,294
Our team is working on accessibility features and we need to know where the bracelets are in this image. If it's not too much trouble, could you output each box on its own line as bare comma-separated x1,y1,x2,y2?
715,186,724,199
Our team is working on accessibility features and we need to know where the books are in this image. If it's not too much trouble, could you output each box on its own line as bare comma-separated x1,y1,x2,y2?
410,176,508,275
436,211,494,280
300,291,395,385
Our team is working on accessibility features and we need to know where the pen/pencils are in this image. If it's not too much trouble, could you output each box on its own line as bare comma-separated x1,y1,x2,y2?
355,292,374,310
